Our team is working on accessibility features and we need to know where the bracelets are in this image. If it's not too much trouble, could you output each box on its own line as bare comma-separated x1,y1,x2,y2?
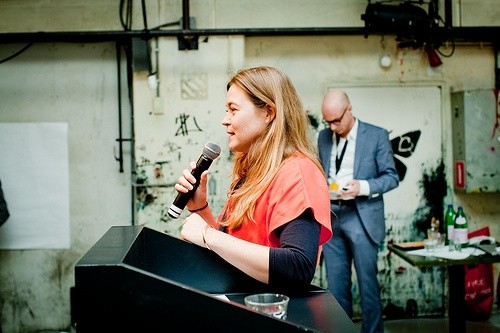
203,224,215,250
188,201,208,212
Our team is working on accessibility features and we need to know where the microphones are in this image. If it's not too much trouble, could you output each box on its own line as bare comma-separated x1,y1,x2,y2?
164,143,222,221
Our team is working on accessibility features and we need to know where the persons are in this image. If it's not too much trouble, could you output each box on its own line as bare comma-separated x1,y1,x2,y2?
317,91,399,333
175,66,333,292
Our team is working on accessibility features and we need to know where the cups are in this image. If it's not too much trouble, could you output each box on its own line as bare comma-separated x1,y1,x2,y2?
428,229,438,239
423,239,437,252
448,238,461,253
244,293,289,321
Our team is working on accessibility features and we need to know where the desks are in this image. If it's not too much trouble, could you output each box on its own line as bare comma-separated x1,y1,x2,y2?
389,244,500,333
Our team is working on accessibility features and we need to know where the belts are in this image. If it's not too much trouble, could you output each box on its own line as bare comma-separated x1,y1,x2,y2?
331,200,355,207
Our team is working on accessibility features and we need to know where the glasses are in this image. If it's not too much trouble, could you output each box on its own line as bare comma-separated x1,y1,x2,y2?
321,106,346,125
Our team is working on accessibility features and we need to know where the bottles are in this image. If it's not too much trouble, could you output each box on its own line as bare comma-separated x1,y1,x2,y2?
454,206,469,247
444,205,456,246
